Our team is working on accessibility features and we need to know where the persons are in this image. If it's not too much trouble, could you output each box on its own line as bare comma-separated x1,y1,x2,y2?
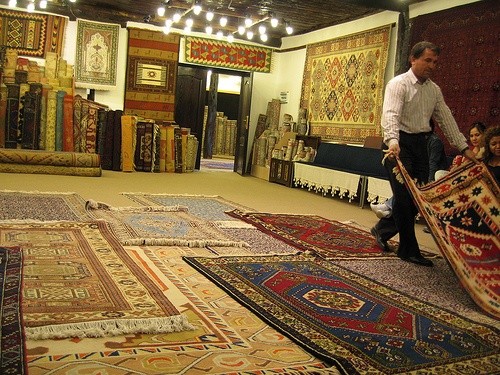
422,122,486,234
372,41,480,268
368,116,448,221
482,125,500,188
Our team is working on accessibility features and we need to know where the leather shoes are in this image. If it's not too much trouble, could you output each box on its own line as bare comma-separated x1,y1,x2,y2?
371,226,389,252
397,249,429,265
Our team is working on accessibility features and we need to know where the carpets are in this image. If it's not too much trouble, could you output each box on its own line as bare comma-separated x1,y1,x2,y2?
0,6,500,375
